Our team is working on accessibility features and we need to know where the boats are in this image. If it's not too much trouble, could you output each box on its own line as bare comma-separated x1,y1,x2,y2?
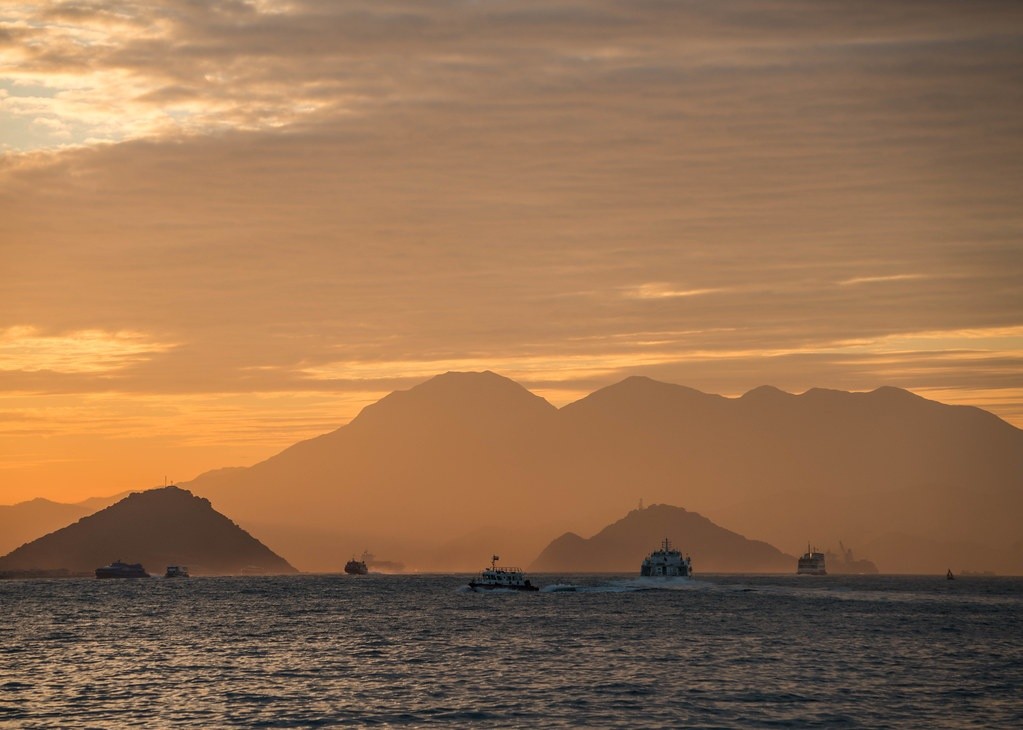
345,561,368,576
94,559,152,579
798,541,827,576
641,539,693,578
947,568,954,581
167,564,190,578
468,554,539,593
